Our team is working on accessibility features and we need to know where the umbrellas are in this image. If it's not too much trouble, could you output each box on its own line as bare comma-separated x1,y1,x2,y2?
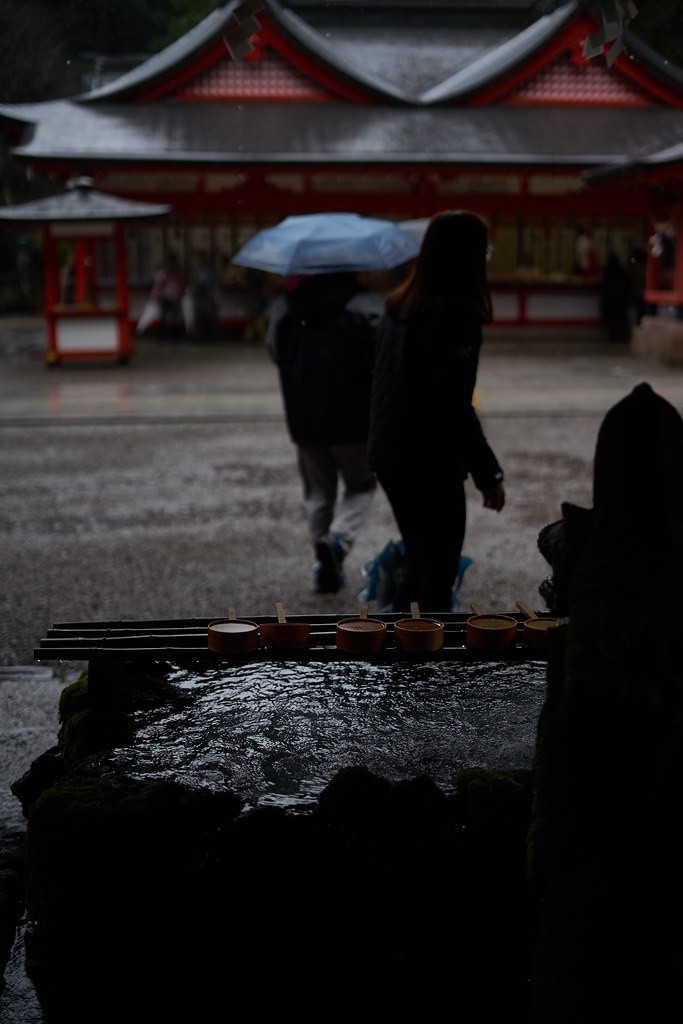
231,211,422,279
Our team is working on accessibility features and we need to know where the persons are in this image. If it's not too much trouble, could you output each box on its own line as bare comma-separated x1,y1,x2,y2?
376,208,513,615
268,265,379,593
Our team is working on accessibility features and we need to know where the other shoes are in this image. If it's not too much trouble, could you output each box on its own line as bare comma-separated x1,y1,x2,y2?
314,535,348,595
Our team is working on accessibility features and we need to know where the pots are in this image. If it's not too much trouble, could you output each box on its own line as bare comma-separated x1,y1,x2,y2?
394,602,444,651
208,607,259,654
467,601,517,651
260,601,314,649
336,605,387,654
516,599,561,646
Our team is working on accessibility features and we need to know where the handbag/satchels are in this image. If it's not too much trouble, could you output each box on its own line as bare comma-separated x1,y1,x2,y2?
358,540,473,606
